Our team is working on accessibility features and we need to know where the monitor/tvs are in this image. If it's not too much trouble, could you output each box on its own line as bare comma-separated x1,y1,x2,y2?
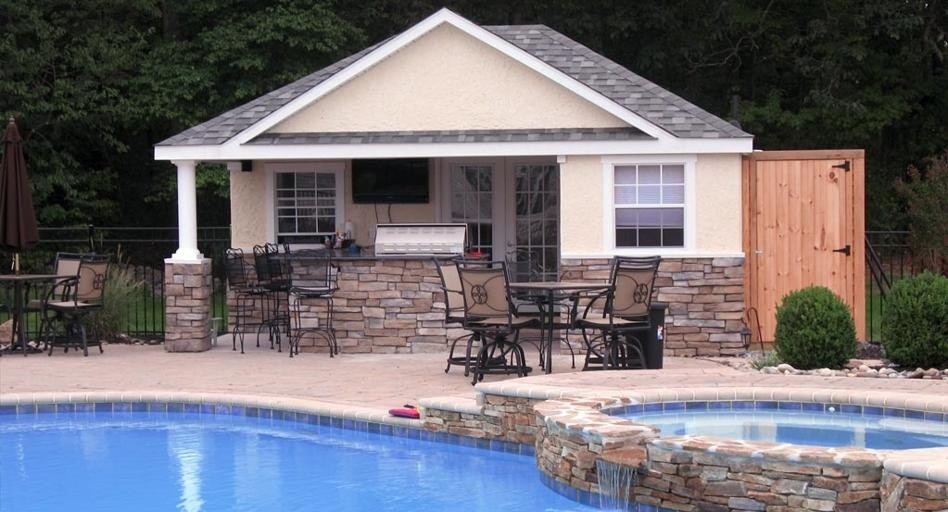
352,159,429,204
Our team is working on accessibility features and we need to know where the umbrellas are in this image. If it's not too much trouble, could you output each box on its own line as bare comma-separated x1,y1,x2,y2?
0,115,44,271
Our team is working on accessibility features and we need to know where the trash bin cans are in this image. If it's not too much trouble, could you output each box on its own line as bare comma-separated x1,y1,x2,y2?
624,300,669,370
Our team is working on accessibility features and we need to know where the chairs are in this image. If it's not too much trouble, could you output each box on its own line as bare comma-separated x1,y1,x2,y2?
223,240,341,358
431,253,663,386
26,251,112,356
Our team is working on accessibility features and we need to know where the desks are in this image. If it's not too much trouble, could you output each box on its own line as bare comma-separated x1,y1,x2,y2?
0,274,81,357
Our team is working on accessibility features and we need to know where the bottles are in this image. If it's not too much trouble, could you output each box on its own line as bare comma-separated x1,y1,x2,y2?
344,219,354,239
